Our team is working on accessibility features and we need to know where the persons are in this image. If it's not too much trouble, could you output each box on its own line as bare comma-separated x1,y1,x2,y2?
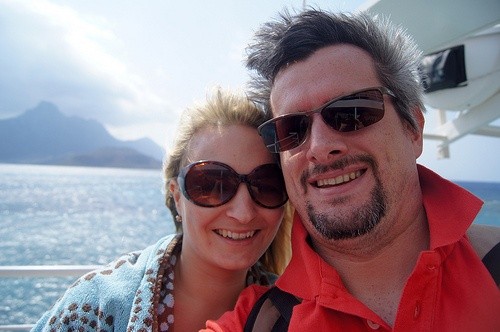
27,90,292,332
199,0,500,332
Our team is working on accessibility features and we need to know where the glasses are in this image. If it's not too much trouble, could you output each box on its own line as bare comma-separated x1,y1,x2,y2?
257,87,398,153
177,160,289,209
